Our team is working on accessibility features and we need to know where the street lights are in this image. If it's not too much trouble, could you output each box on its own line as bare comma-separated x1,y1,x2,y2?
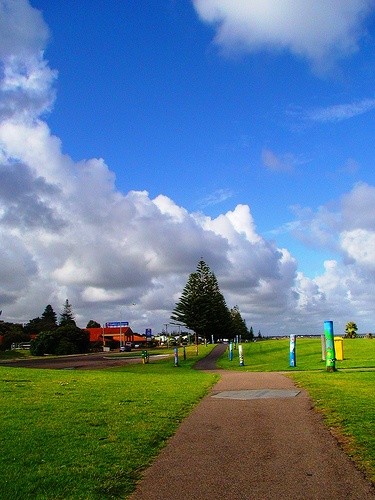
164,323,169,346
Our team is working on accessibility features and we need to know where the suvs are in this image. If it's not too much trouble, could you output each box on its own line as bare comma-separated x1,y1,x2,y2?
120,346,130,352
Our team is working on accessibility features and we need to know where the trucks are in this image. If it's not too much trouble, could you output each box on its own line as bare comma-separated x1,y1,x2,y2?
125,342,135,347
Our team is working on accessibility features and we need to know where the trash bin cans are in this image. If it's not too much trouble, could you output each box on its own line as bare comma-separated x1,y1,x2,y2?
139,349,149,365
